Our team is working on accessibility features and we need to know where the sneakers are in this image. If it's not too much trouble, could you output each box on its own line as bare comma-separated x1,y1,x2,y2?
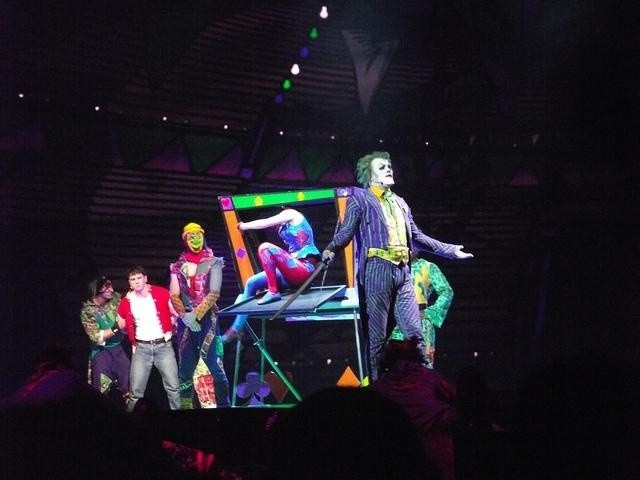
257,291,281,305
222,334,232,344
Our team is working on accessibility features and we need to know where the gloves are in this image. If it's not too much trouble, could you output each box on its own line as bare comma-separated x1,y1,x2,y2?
456,245,474,259
322,249,335,263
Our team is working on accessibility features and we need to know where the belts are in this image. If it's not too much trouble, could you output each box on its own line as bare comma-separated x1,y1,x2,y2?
135,337,166,344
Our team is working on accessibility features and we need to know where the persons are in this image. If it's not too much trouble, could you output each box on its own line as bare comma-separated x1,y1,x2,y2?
367,335,460,480
2,342,86,414
237,206,322,305
79,273,131,417
169,222,232,410
320,151,475,385
115,266,182,420
385,249,454,371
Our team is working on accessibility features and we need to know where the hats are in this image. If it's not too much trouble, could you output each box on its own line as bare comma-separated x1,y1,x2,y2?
182,223,205,238
